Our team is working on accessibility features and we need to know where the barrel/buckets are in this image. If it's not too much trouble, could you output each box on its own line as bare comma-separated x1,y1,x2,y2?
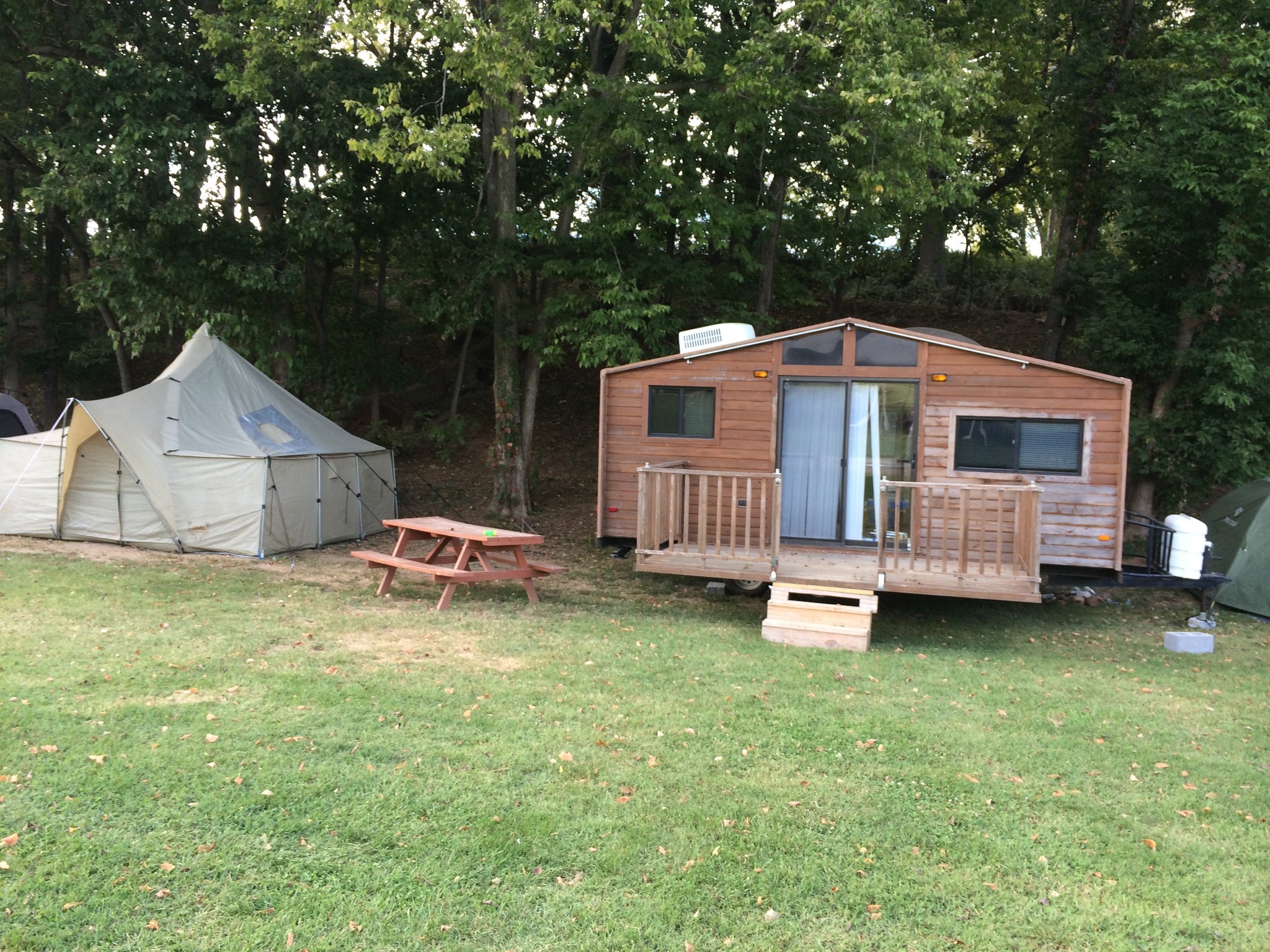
1160,513,1208,580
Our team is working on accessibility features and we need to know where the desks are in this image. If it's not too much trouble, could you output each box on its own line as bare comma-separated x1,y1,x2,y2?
375,516,544,609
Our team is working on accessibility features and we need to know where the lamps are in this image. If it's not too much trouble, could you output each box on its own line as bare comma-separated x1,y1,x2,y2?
931,372,947,382
753,369,770,378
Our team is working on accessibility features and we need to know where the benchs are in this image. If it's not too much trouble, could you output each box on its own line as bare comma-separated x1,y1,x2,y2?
350,546,569,584
